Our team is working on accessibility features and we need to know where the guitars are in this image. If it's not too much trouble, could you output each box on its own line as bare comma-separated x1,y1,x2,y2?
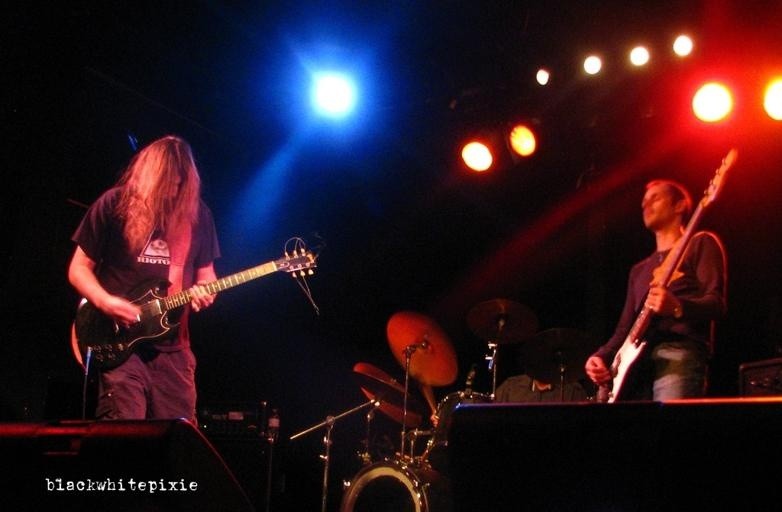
598,147,737,403
74,247,317,372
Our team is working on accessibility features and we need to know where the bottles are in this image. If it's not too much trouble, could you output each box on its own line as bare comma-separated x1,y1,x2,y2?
258,400,281,445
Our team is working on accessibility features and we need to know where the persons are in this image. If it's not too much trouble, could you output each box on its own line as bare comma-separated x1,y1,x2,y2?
63,135,222,432
583,178,730,403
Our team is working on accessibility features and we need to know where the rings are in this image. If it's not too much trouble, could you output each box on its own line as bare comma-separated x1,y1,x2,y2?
649,304,655,311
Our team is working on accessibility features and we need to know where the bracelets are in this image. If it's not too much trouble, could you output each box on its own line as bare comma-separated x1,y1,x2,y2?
671,301,682,317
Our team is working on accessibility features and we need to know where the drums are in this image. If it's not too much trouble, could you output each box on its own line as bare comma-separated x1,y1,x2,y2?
340,452,454,510
428,392,494,475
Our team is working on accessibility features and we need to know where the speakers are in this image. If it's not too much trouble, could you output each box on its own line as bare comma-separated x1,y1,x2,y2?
430,398,782,512
1,421,249,511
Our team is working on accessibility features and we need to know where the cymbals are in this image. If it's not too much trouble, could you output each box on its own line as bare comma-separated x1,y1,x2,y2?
351,362,423,428
520,328,600,384
387,311,459,386
467,297,539,344
350,371,430,417
